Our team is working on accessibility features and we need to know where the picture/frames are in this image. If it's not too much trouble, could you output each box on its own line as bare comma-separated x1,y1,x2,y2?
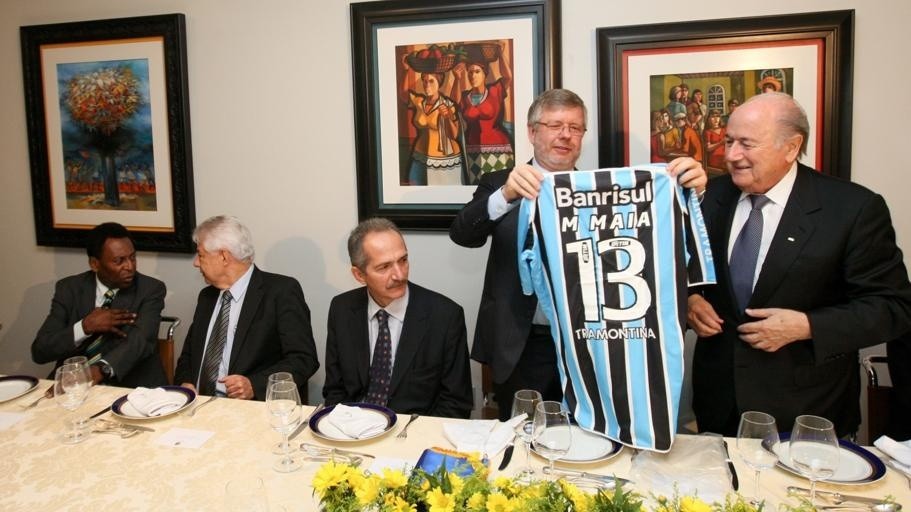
18,12,198,256
595,8,857,181
348,0,564,233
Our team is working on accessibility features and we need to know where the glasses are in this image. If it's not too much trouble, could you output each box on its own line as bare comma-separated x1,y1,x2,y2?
535,120,583,133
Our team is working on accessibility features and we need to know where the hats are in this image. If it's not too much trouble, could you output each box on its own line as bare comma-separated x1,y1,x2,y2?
673,112,686,121
671,86,683,99
757,76,782,91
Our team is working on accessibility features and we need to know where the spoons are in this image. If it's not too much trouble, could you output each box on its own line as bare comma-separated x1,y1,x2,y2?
822,503,895,512
575,481,616,489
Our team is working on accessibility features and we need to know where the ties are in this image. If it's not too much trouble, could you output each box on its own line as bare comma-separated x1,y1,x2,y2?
198,290,233,397
363,309,393,407
727,195,772,316
84,290,114,366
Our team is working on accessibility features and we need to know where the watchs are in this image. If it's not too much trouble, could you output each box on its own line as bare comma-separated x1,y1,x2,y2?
90,361,112,382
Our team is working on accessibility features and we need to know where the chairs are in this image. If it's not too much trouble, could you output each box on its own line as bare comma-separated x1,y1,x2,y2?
158,316,181,384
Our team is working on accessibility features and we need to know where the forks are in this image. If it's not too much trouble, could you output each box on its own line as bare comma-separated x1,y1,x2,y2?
397,413,419,438
18,384,54,410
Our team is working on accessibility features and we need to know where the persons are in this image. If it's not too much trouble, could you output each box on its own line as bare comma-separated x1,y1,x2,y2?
398,53,465,187
666,87,687,143
685,90,911,446
31,223,166,398
679,83,692,107
660,108,681,151
450,89,707,425
703,110,728,170
757,78,780,94
687,89,707,134
173,216,319,406
449,42,511,187
674,112,702,164
720,97,739,128
649,111,673,163
322,218,473,419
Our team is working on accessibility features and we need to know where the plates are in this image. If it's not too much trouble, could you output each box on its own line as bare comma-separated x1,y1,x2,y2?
111,387,198,420
310,403,398,443
761,433,887,486
0,374,40,403
530,424,624,464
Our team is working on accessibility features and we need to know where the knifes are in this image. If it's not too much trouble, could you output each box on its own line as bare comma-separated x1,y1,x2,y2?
724,442,739,492
498,443,515,470
543,467,630,483
788,487,902,511
287,404,324,442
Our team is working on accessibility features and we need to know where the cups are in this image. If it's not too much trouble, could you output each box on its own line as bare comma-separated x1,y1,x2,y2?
532,401,572,492
266,383,303,472
788,415,839,505
266,372,293,455
510,389,546,474
54,364,89,444
65,356,91,429
736,411,781,512
